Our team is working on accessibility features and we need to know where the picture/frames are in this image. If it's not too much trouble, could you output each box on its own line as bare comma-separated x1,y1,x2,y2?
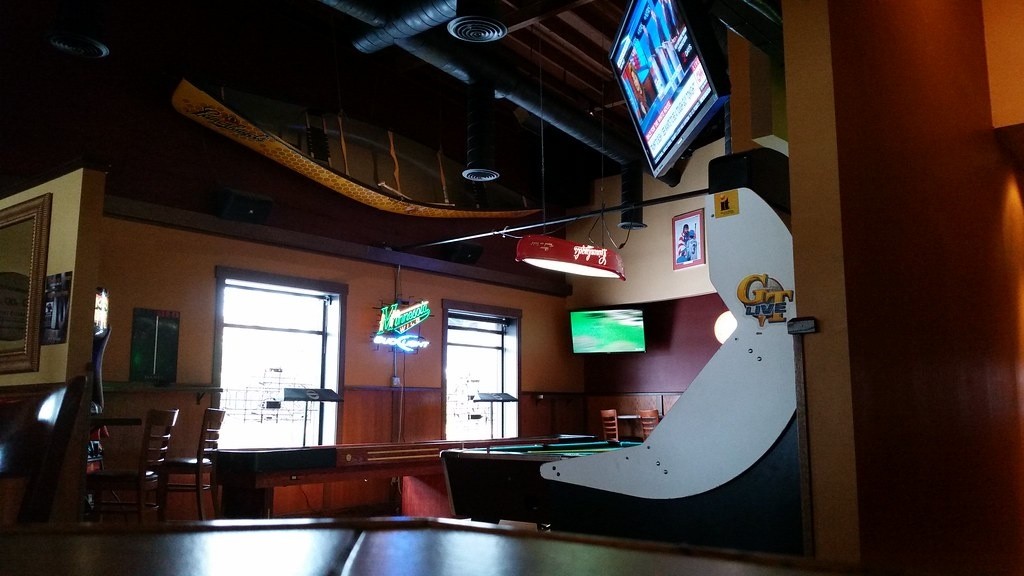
672,207,706,270
0,192,52,375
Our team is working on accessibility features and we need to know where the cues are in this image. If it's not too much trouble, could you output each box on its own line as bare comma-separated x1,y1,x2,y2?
528,449,611,454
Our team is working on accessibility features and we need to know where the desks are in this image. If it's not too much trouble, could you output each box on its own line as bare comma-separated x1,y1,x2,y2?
617,413,664,419
0,517,886,576
439,441,645,531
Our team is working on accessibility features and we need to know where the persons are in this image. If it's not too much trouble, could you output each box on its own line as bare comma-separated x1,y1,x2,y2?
677,224,697,262
628,64,652,120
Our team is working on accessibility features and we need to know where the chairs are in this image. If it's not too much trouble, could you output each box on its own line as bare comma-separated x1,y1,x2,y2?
639,409,660,442
600,408,643,442
147,408,225,521
84,408,180,522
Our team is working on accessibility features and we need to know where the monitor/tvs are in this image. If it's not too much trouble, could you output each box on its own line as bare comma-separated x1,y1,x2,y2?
570,309,647,354
608,0,733,177
